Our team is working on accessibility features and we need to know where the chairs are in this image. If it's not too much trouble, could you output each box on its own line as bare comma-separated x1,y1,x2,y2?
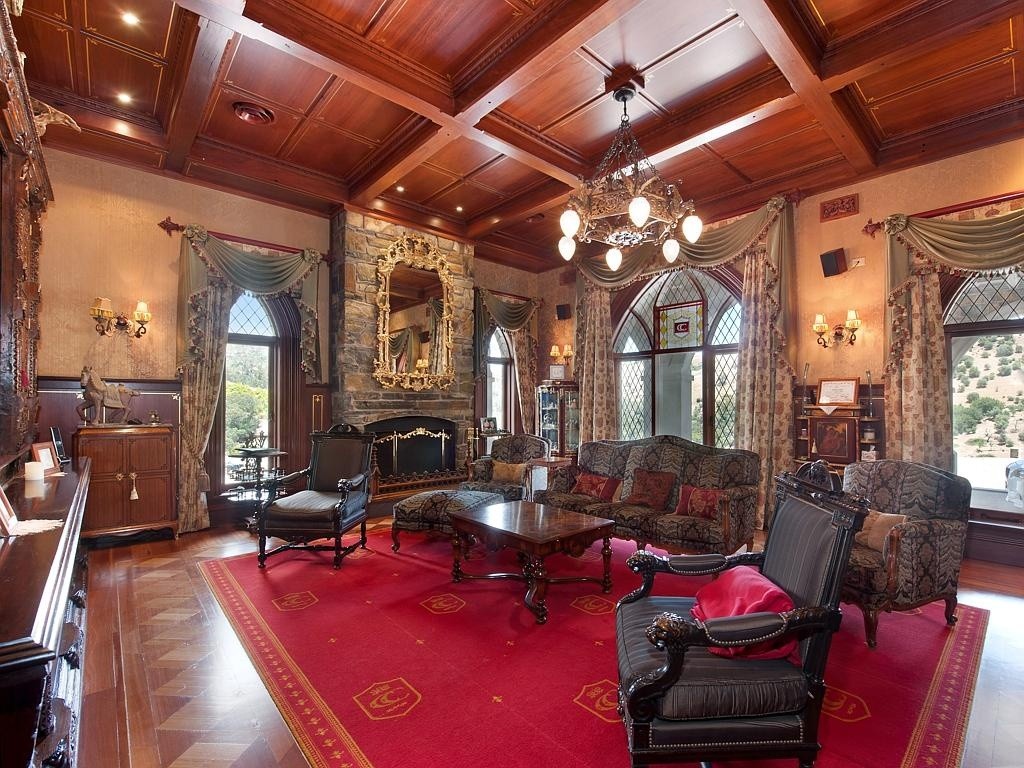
829,455,974,650
458,432,554,502
610,473,870,767
255,423,379,572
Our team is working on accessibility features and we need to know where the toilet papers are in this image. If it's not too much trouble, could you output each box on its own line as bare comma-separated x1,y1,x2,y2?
23,462,45,480
23,480,45,497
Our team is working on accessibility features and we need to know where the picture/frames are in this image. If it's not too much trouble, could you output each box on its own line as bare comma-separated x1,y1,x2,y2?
0,487,19,573
814,376,861,408
31,441,62,476
479,416,498,432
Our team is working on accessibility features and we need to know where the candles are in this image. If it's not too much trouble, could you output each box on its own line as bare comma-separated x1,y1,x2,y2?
25,460,45,480
25,480,44,498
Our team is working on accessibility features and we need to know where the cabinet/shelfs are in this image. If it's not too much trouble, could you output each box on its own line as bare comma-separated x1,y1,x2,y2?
69,423,184,541
793,381,885,468
534,376,582,460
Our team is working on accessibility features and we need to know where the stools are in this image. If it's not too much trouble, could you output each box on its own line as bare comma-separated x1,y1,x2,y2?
389,488,502,561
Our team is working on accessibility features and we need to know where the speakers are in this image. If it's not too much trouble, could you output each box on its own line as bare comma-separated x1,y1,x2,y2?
557,304,570,320
819,247,846,278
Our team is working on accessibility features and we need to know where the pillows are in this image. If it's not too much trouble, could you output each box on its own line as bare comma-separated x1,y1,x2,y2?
622,466,676,513
489,457,528,484
853,501,909,552
568,469,622,502
673,484,729,521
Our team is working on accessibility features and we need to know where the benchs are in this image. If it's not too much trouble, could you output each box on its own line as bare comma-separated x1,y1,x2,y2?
545,433,764,559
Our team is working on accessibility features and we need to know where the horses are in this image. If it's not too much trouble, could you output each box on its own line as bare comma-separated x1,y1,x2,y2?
76,366,139,426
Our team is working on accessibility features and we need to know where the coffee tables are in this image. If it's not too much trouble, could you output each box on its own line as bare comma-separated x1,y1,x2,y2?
440,499,616,626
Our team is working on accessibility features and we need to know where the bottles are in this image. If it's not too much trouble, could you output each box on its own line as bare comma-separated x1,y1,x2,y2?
864,425,875,441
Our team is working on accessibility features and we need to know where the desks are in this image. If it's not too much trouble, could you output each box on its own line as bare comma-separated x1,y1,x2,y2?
225,442,289,533
525,452,575,502
0,451,97,768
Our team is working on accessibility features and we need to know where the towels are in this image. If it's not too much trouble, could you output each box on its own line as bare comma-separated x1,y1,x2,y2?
688,565,798,660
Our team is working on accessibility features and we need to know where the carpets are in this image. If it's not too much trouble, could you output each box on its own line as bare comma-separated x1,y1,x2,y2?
195,516,993,768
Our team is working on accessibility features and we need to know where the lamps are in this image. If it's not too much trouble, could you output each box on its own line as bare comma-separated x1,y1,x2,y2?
550,343,575,367
812,308,862,349
558,80,706,275
87,295,154,339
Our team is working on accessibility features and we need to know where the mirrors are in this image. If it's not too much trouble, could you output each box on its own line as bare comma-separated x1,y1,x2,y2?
370,231,460,392
